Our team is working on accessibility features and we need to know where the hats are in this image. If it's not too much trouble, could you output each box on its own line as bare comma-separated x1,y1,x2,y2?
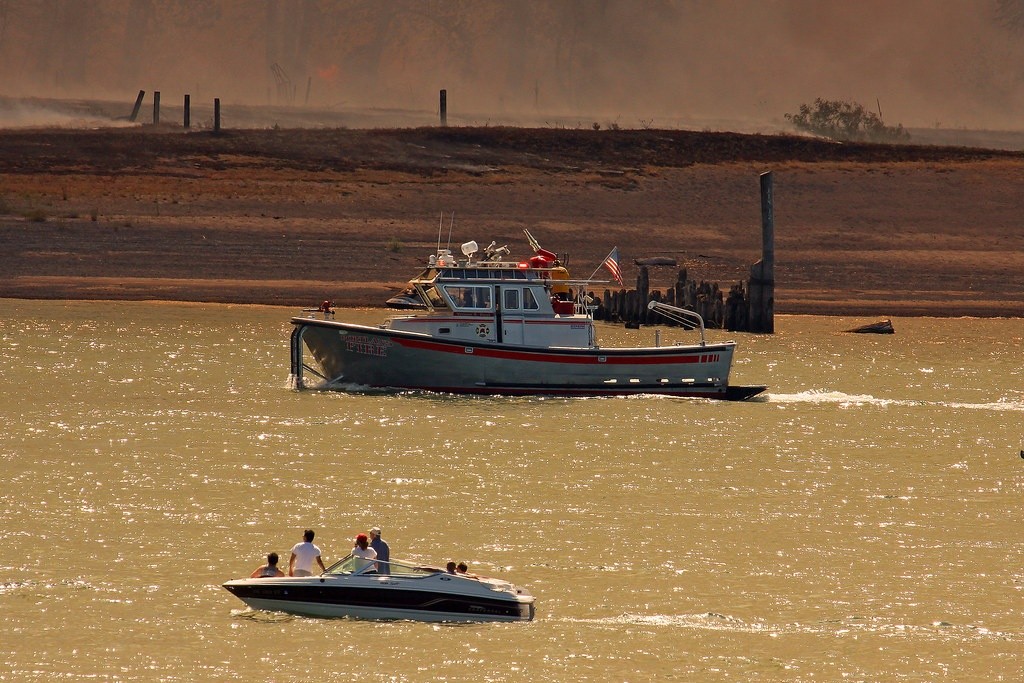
367,527,381,535
355,534,367,542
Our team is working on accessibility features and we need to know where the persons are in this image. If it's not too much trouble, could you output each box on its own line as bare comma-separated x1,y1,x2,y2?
350,525,391,575
250,552,285,579
483,295,499,310
551,259,571,301
445,562,469,576
461,292,473,307
288,530,326,577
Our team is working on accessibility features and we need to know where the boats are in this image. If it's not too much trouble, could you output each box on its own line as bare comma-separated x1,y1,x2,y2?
222,551,538,623
288,210,771,403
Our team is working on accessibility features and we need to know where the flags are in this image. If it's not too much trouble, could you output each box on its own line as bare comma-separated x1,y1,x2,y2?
604,248,624,286
